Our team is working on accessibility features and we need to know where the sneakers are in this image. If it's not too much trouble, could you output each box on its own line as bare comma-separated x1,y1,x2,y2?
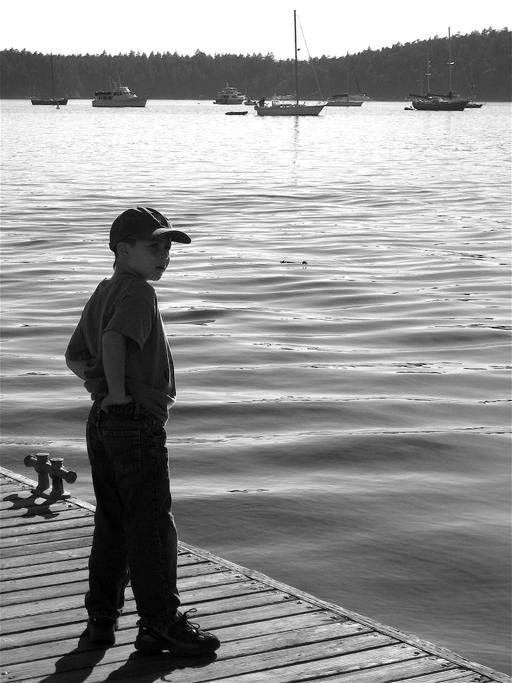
135,609,221,657
87,615,120,644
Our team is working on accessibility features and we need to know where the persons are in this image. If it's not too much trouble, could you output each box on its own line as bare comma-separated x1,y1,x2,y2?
62,207,222,659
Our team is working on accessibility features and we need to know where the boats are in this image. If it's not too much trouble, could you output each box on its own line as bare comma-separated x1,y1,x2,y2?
92,86,150,107
213,86,244,104
327,94,364,106
30,96,68,104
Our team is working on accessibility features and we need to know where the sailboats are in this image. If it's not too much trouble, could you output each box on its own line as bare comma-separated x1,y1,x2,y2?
254,10,327,116
413,28,483,111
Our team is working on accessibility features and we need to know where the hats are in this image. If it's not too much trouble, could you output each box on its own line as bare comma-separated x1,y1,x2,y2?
109,206,191,251
